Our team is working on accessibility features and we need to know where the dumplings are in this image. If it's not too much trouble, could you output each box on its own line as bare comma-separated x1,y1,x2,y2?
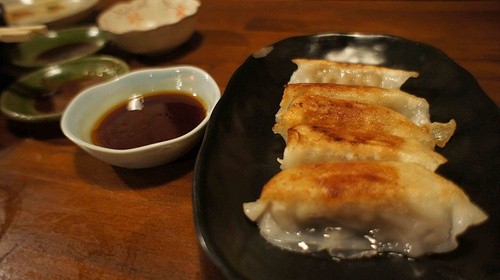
240,56,488,259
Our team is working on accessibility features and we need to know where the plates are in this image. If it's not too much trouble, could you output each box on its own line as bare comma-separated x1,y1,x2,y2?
2,0,99,25
0,56,130,121
6,26,109,67
191,32,500,280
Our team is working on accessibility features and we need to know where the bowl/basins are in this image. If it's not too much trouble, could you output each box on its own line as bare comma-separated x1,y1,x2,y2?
60,65,221,168
96,0,201,53
